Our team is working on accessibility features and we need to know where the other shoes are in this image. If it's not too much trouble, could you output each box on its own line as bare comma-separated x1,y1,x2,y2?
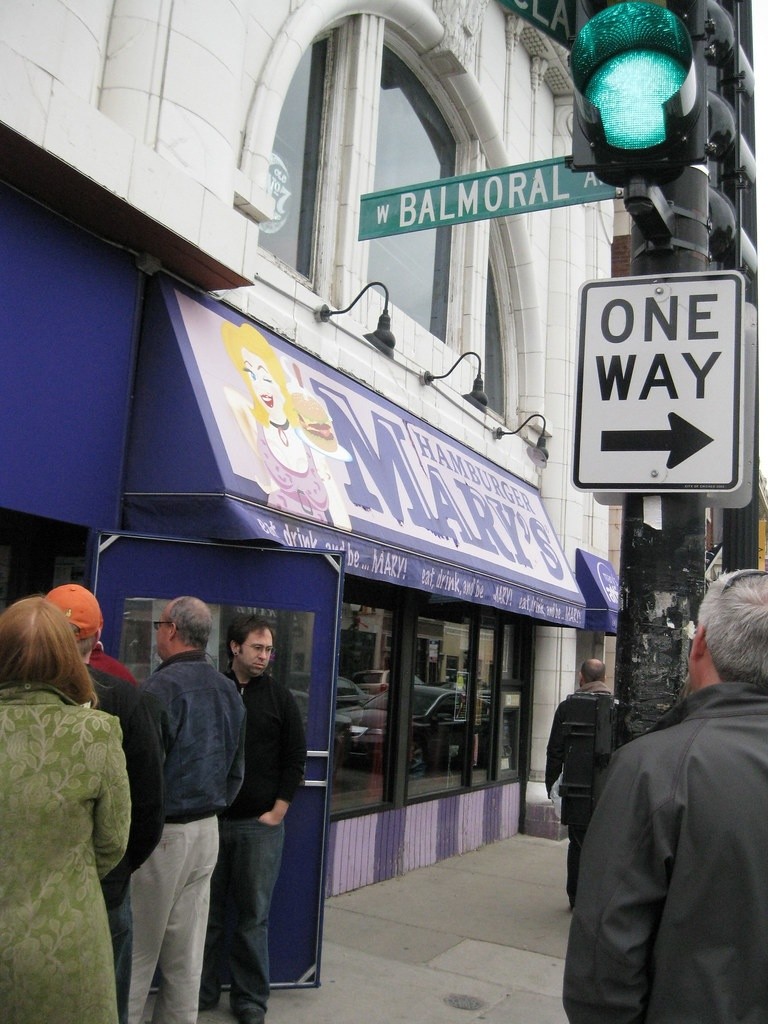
234,1009,264,1024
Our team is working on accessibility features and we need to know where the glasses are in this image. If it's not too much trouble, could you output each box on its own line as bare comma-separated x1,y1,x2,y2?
243,642,276,655
153,620,179,630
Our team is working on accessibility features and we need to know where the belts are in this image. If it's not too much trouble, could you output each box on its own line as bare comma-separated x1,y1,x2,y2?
166,811,217,825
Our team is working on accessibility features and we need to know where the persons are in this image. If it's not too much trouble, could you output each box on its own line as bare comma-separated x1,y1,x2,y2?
562,568,768,1024
544,658,613,908
44,583,171,1024
198,615,308,1024
127,597,247,1024
0,596,132,1024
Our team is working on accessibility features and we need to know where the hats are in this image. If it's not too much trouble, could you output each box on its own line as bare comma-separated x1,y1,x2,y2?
44,584,103,640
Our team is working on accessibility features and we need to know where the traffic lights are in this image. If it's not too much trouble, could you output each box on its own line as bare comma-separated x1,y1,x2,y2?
566,0,701,178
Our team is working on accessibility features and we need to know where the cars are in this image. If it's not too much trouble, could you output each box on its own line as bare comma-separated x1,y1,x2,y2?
284,672,375,710
351,669,427,696
439,676,520,714
342,687,494,770
286,688,353,783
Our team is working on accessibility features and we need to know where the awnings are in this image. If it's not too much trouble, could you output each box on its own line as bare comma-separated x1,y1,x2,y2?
122,270,586,630
575,549,620,636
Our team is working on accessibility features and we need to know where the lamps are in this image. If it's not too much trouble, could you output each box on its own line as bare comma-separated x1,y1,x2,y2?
316,282,396,361
420,352,488,414
493,413,549,469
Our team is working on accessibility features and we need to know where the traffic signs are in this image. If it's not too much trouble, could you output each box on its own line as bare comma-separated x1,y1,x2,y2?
569,270,748,495
